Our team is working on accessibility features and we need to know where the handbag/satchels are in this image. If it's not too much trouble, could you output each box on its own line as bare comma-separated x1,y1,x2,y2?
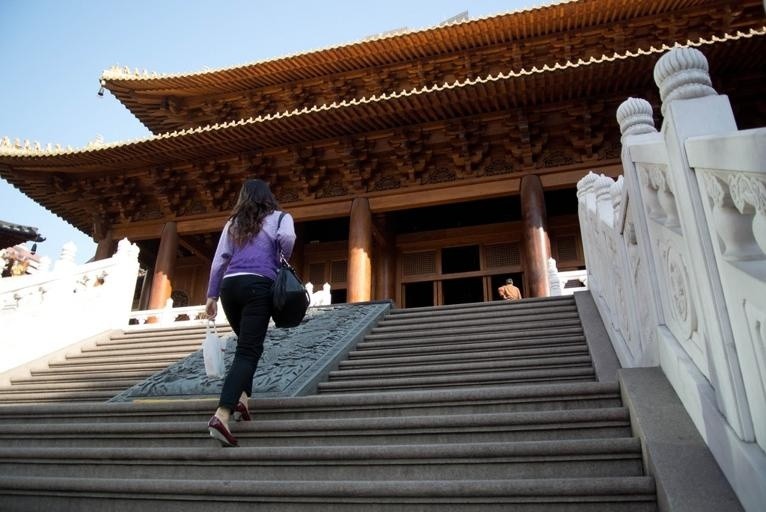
202,317,229,381
269,265,311,330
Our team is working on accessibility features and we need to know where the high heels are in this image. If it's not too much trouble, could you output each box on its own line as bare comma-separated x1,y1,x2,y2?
231,400,253,422
207,415,239,448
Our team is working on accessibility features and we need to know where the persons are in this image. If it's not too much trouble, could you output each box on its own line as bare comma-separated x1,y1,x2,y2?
498,279,522,300
204,178,298,448
